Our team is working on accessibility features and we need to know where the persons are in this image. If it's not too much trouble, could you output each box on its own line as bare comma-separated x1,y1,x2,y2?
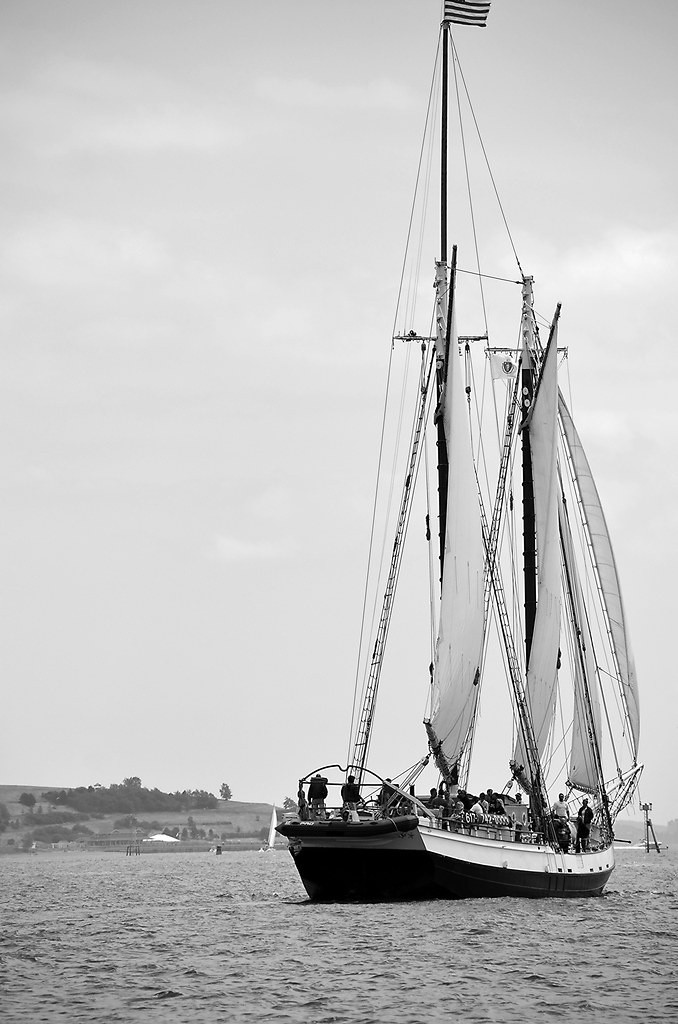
308,774,328,814
575,816,589,853
429,788,483,829
577,799,593,852
552,793,570,822
479,789,506,815
340,775,359,808
557,818,571,839
380,778,392,795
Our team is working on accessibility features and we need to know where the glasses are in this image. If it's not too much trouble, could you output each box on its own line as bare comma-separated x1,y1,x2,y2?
456,804,460,805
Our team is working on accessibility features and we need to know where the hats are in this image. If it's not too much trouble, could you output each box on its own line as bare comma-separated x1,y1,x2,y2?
515,793,522,800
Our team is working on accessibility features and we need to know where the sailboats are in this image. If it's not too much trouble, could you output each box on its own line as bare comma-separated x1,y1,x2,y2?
256,804,278,853
284,0,646,910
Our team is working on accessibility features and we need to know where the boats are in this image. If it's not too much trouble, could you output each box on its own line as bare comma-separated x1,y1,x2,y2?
636,837,663,848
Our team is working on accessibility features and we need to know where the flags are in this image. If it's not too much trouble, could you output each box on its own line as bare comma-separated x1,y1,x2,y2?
443,0,491,28
491,355,517,379
